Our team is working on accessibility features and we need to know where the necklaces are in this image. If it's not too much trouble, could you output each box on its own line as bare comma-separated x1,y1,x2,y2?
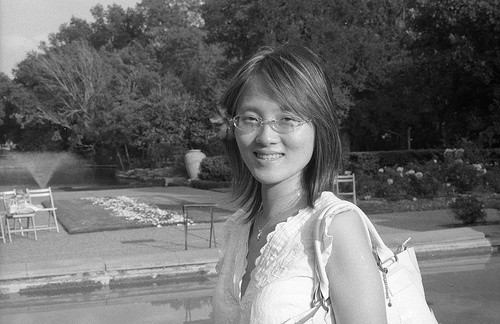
256,188,303,240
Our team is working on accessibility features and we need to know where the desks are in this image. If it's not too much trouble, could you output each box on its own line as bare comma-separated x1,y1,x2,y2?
181,201,218,250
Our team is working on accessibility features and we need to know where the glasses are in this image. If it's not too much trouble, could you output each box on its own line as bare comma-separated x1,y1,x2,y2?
230,114,312,132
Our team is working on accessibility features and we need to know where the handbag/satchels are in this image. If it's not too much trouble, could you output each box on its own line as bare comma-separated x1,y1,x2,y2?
282,200,439,324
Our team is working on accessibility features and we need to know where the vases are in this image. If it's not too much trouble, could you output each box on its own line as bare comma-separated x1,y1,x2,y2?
184,149,206,182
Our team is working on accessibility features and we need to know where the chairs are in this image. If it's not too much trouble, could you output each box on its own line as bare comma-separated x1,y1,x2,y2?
0,187,60,243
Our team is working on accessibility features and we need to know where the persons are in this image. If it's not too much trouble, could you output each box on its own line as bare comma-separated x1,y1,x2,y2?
213,44,387,324
